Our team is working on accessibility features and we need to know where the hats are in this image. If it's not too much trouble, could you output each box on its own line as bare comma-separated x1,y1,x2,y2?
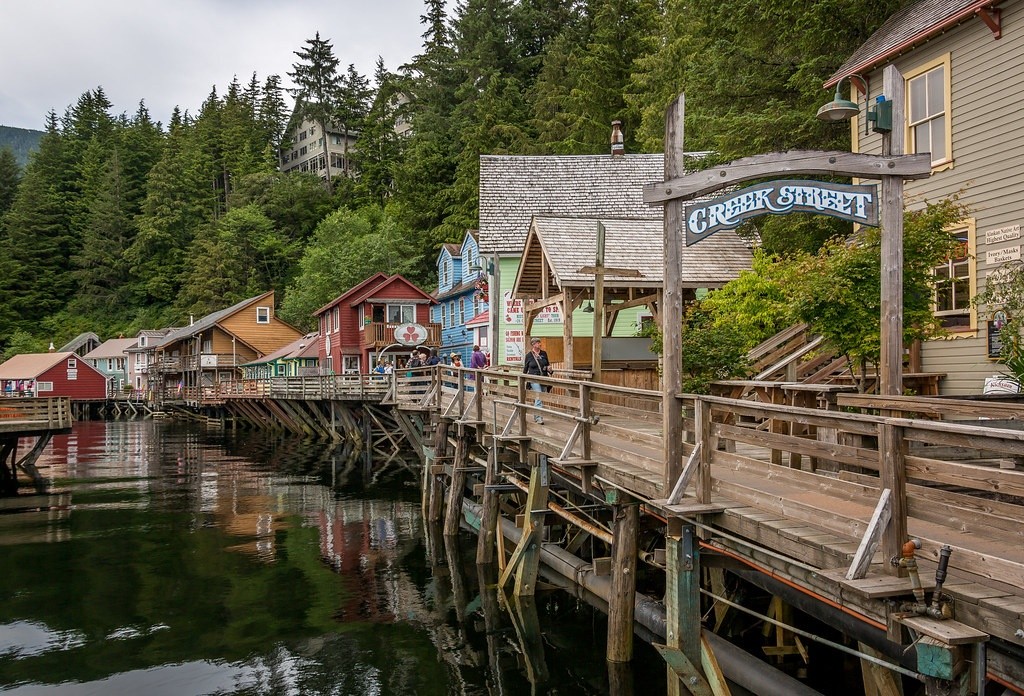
450,352,457,358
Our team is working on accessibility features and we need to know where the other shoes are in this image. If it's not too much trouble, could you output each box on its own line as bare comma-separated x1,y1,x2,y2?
537,418,544,425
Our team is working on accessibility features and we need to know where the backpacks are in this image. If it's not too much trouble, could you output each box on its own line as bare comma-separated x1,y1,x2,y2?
411,358,422,375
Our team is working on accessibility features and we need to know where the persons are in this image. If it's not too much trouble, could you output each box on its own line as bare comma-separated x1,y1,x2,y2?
469,344,487,394
371,349,465,403
523,338,552,425
